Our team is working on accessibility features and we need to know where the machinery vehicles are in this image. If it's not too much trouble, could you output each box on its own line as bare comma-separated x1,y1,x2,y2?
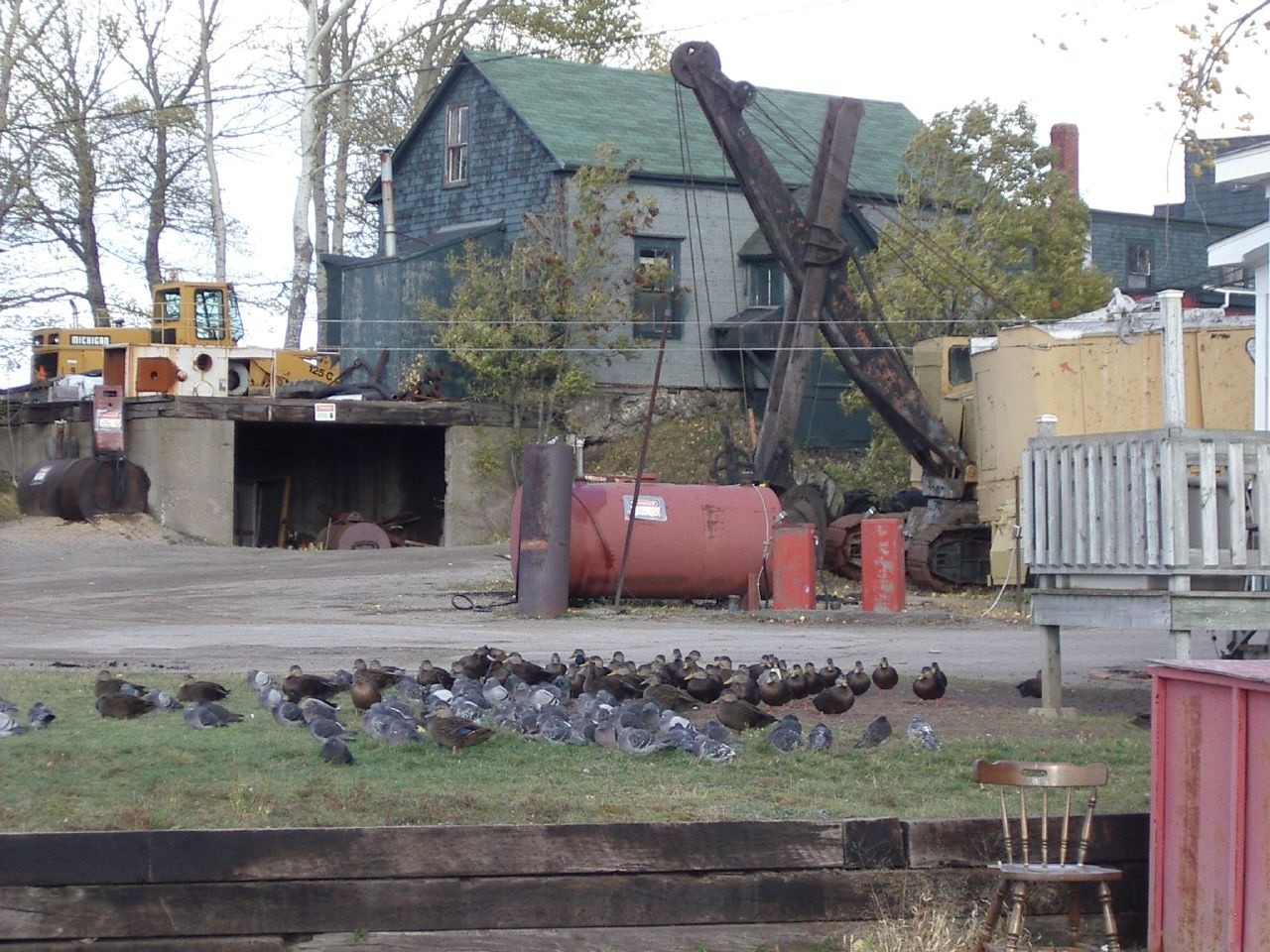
30,280,348,397
670,34,1263,597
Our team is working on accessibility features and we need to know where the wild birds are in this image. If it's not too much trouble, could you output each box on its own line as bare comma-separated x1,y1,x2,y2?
93,669,243,730
1015,670,1042,699
0,697,55,738
247,644,947,763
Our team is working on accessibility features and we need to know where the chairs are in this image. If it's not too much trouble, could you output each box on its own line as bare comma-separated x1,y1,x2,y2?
971,758,1124,952
